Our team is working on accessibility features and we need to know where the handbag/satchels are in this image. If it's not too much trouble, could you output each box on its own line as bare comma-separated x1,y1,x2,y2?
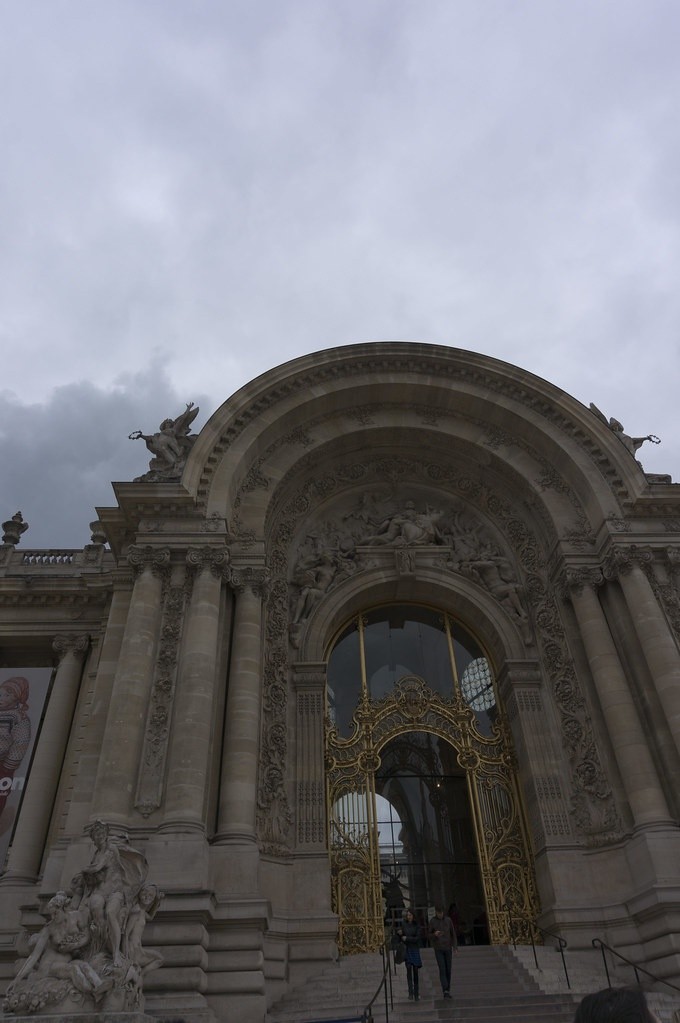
394,942,409,964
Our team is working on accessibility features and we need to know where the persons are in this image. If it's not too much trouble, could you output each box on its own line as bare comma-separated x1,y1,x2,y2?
5,819,161,1002
574,986,662,1022
402,500,417,522
392,907,421,1001
475,546,529,618
368,513,412,547
427,904,458,1000
289,550,337,624
0,676,31,818
590,402,649,458
140,402,194,465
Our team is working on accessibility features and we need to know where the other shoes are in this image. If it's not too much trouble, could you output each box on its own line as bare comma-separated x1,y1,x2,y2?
444,993,451,1000
415,993,420,1001
407,993,414,1000
